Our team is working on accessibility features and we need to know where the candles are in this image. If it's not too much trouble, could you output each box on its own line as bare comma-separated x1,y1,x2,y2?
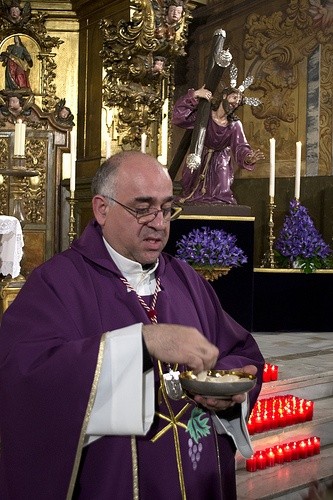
70,131,76,191
141,132,147,153
161,97,168,164
246,436,321,472
14,118,26,155
295,141,302,197
263,363,279,382
269,137,276,197
247,395,314,435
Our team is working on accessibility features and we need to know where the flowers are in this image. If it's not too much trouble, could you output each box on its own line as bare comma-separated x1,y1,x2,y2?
176,226,247,266
275,199,333,274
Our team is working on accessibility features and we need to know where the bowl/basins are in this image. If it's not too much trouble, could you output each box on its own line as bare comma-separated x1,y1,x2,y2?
179,370,257,400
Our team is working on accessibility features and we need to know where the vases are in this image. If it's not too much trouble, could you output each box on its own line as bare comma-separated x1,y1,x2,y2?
292,261,314,269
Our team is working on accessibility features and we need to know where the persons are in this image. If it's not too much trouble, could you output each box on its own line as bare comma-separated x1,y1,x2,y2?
0,0,192,125
0,149,264,500
172,88,265,204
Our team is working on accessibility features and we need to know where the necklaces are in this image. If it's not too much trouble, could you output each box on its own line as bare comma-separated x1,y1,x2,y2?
118,276,184,401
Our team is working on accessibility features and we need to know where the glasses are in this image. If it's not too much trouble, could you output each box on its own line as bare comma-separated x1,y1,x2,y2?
101,195,183,225
